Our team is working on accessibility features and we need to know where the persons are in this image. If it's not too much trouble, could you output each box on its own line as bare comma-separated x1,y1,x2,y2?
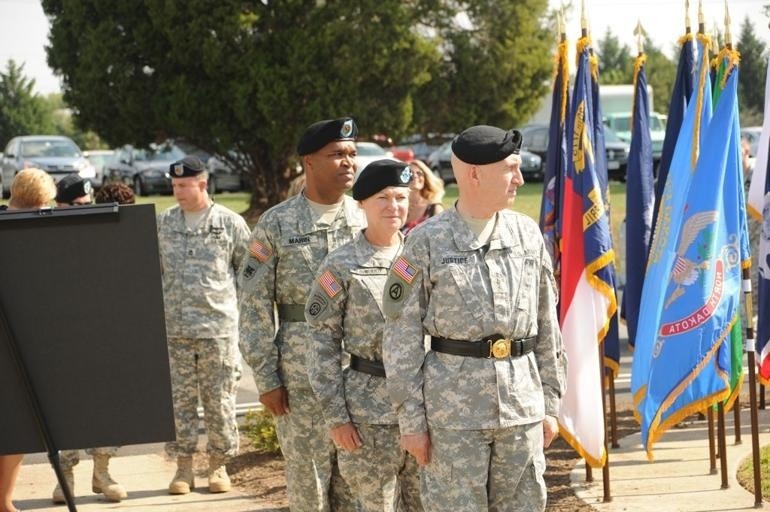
399,160,447,239
54,172,94,207
0,167,58,512
740,130,762,354
304,158,416,512
156,156,250,494
381,124,568,512
43,445,138,504
93,181,136,208
237,117,369,512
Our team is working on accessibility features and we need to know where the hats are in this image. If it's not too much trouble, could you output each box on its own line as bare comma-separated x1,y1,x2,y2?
450,125,523,166
296,116,358,155
54,173,90,202
351,159,412,200
169,157,204,179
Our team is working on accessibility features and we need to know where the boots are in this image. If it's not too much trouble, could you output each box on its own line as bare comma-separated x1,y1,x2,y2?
168,457,195,495
91,455,128,500
52,468,75,503
207,457,231,492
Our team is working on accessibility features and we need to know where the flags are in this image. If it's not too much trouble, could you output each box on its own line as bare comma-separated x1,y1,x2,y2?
557,36,619,468
620,54,657,356
652,32,696,239
747,60,770,390
540,40,571,275
644,45,755,459
590,51,620,391
633,32,715,423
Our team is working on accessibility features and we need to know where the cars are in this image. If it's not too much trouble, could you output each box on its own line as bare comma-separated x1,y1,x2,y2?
191,151,250,194
352,141,400,185
426,137,542,186
102,142,187,196
1,135,96,192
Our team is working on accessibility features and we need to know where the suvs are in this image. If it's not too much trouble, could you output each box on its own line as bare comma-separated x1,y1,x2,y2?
521,121,629,185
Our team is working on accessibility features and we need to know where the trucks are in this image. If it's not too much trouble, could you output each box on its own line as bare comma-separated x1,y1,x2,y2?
531,83,666,162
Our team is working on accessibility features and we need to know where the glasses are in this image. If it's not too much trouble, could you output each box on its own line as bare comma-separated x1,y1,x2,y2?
410,170,423,176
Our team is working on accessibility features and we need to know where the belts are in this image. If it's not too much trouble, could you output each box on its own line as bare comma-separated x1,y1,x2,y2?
348,354,386,378
430,336,537,358
277,304,306,322
